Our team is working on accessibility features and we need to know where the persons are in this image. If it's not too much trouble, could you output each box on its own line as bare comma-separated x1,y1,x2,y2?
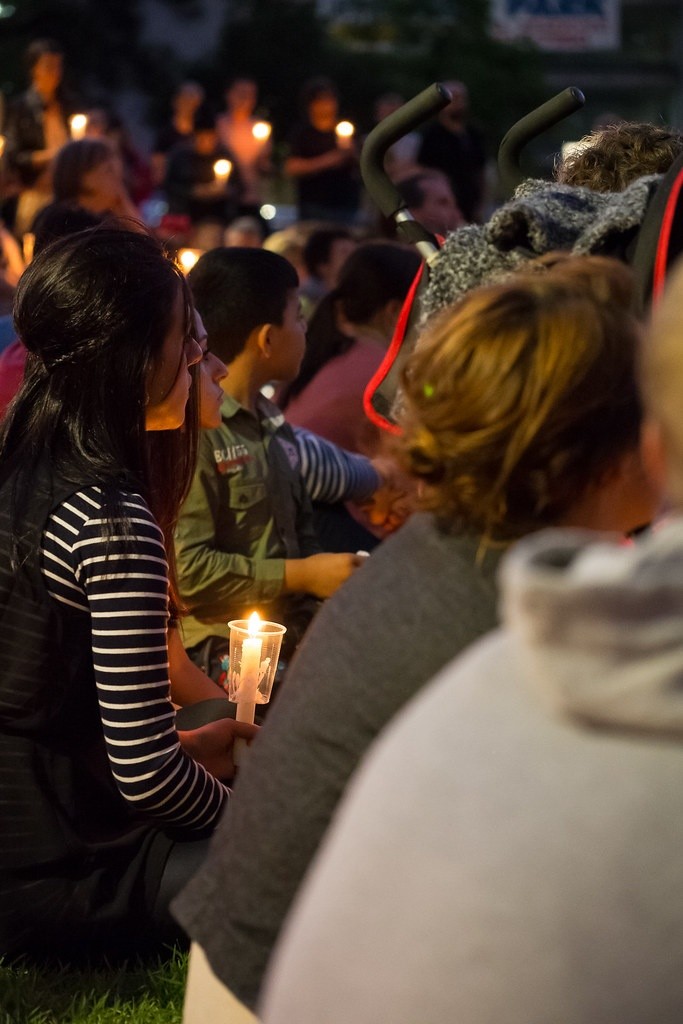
0,45,683,1024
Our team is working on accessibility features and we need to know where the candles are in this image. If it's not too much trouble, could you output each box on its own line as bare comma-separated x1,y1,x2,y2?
233,610,265,766
68,106,356,282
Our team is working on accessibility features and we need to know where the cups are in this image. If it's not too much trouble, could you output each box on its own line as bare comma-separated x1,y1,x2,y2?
228,620,287,703
